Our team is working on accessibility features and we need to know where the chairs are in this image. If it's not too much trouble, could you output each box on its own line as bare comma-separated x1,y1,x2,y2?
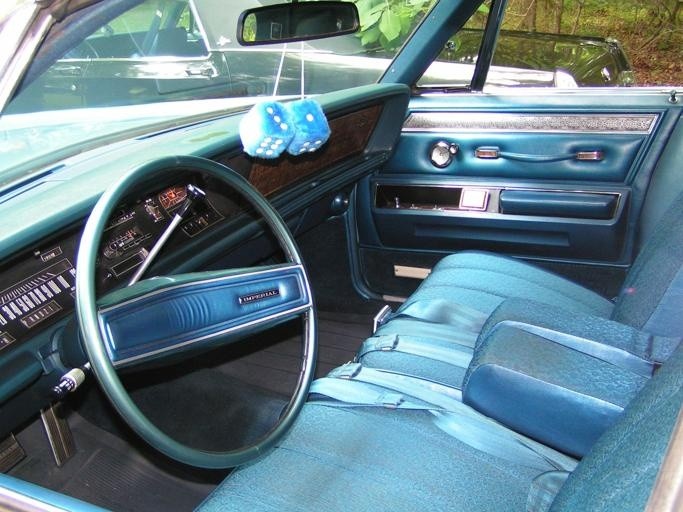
186,334,681,512
355,111,680,372
